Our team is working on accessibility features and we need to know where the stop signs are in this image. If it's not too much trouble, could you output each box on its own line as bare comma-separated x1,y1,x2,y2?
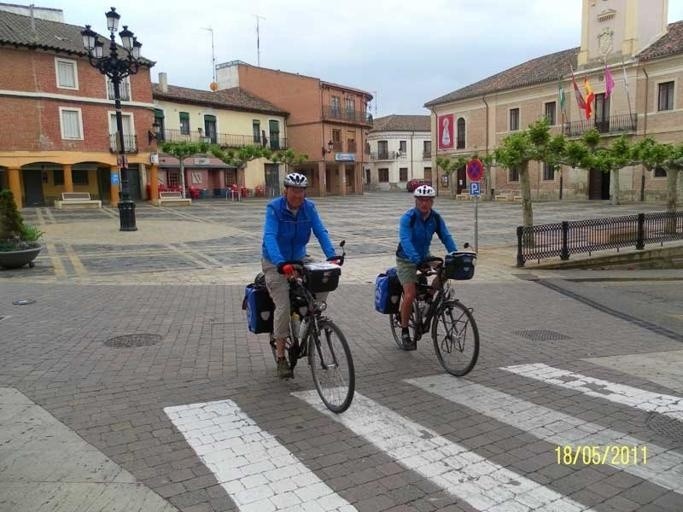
466,160,482,181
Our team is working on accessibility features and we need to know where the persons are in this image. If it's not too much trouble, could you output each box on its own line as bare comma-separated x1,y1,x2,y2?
261,172,337,378
395,184,458,350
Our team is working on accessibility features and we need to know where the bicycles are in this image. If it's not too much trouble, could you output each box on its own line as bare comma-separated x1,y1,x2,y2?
251,255,355,413
385,251,479,377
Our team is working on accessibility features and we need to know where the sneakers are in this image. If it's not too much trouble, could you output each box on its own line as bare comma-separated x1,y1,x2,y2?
276,357,294,380
401,329,416,350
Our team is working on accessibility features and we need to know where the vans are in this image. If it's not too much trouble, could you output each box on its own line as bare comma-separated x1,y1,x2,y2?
407,178,431,190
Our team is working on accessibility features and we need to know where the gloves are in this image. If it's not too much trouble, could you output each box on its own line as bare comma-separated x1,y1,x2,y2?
414,262,432,276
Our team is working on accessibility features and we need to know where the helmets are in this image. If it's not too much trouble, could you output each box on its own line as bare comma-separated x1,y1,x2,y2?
284,172,309,188
413,184,436,198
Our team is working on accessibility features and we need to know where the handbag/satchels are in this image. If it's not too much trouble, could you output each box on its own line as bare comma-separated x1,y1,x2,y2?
241,282,275,334
374,269,401,315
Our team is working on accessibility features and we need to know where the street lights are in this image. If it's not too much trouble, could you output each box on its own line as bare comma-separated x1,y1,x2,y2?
79,6,140,230
249,12,268,66
201,26,217,84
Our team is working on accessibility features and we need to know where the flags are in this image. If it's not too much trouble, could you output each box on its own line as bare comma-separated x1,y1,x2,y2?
603,64,615,99
573,73,586,110
558,82,566,112
584,81,594,120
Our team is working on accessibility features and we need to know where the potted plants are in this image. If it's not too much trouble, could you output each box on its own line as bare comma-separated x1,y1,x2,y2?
0,223,45,269
